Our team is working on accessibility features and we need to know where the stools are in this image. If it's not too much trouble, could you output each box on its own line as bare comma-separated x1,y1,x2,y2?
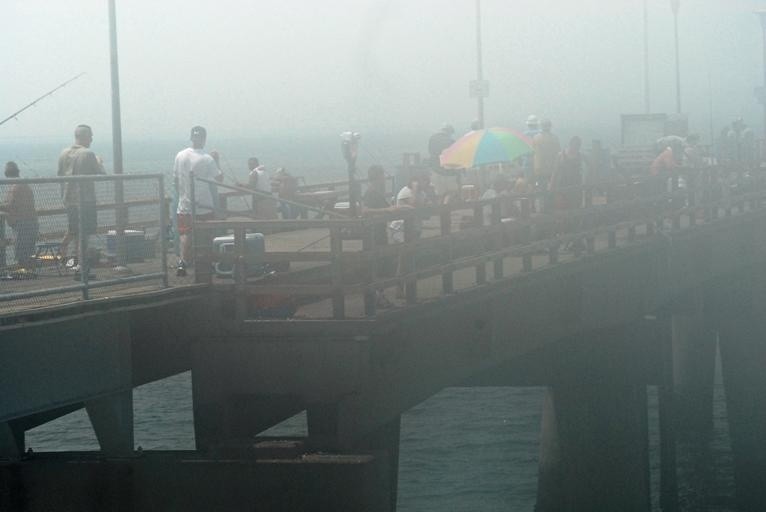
31,243,67,276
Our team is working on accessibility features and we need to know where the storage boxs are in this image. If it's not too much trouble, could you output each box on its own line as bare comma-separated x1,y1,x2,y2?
333,201,351,216
212,233,267,278
105,228,145,263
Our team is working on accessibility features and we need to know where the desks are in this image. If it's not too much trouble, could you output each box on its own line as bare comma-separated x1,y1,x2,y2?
297,189,348,219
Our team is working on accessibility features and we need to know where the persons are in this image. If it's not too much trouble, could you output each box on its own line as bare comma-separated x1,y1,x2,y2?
352,116,615,309
642,115,765,235
55,124,107,278
170,125,224,275
237,158,272,235
0,160,37,275
270,167,299,232
